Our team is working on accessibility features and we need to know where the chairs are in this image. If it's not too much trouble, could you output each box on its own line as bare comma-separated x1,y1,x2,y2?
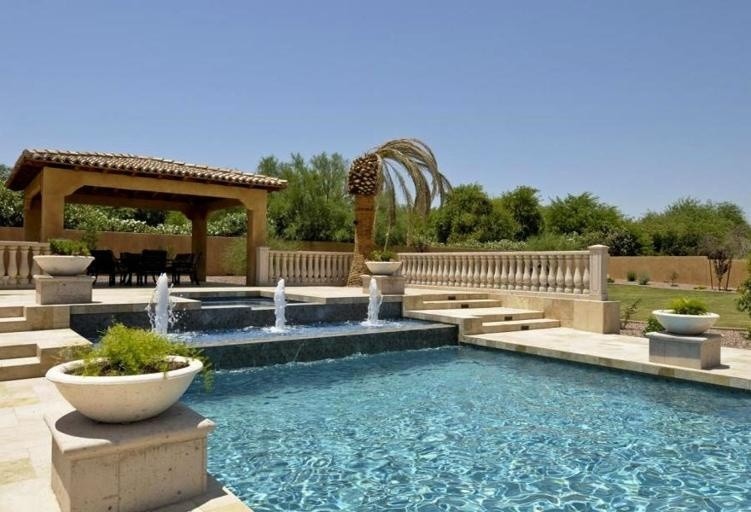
87,248,203,288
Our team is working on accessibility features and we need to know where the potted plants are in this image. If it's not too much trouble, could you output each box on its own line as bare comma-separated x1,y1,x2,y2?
32,236,97,276
364,249,405,275
43,319,217,424
652,297,723,335
